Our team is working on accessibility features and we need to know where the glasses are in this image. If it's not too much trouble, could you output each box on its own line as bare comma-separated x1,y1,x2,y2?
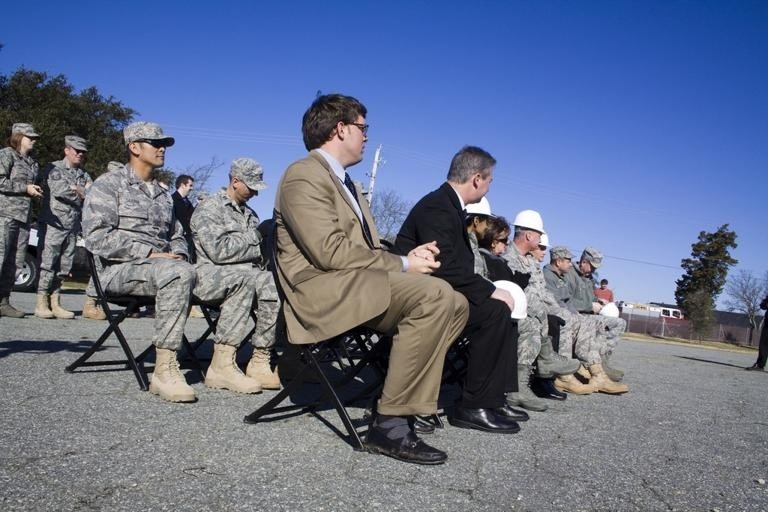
133,139,167,149
345,119,370,135
493,237,510,248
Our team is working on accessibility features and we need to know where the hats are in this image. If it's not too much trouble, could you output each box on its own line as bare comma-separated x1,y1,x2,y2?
11,123,40,139
229,156,268,192
122,121,175,148
107,159,124,172
581,247,605,269
551,246,577,261
63,134,88,154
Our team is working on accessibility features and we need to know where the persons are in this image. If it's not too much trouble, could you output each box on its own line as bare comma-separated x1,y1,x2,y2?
745,293,768,370
34,135,93,319
502,209,629,396
82,161,124,319
190,157,280,389
542,246,624,381
479,216,567,402
593,278,614,303
568,248,626,358
530,231,550,262
393,145,529,435
465,196,581,412
272,94,470,465
0,123,44,319
82,120,261,402
171,174,205,318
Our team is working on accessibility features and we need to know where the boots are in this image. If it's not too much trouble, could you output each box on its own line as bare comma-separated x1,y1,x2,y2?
536,338,581,378
149,348,196,404
245,347,282,390
81,297,107,320
599,353,625,382
587,364,629,394
505,363,549,411
49,294,74,320
188,305,205,320
0,296,26,318
32,293,53,319
203,344,264,395
551,374,594,395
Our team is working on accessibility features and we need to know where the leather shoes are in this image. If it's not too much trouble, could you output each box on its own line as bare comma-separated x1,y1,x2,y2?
408,416,436,434
529,379,568,401
363,425,449,464
491,395,529,422
450,404,521,434
746,363,765,372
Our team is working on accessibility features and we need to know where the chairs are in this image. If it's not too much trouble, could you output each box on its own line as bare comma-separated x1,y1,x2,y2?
243,220,443,453
65,251,205,393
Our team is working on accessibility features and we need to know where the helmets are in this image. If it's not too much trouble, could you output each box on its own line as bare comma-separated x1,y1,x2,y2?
537,234,552,249
509,209,547,238
598,301,619,318
465,195,494,219
